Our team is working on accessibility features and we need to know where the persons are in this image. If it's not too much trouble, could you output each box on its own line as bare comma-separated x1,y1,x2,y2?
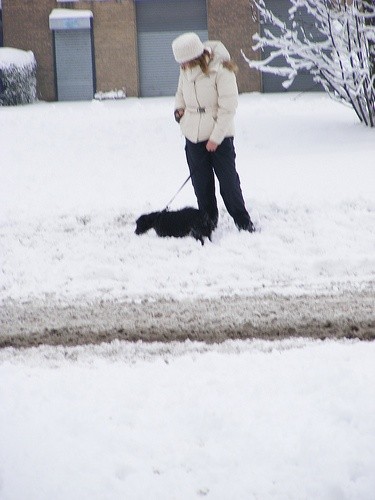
172,32,256,232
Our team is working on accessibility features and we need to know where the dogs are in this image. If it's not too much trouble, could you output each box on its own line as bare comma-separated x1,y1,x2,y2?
134,205,215,246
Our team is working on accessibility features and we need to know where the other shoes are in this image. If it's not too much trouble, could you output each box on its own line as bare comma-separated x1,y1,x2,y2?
236,222,255,232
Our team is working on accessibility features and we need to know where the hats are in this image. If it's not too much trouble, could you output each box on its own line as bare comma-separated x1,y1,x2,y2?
172,32,206,63
